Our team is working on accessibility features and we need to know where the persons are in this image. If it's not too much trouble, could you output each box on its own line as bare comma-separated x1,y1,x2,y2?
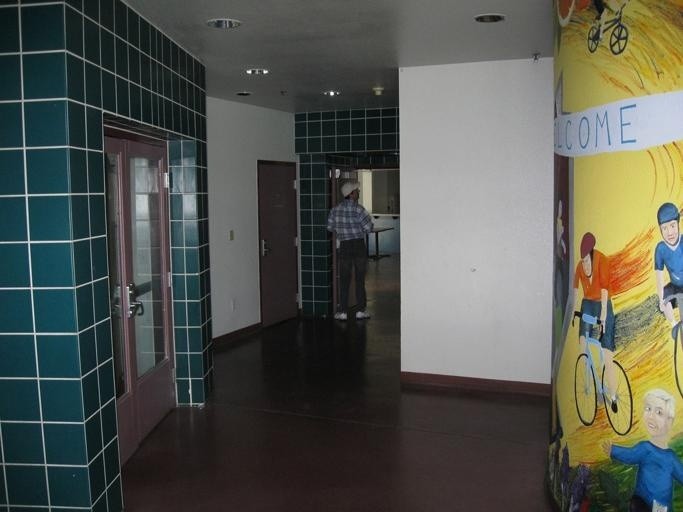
326,181,374,321
602,389,683,512
571,232,617,413
654,202,683,347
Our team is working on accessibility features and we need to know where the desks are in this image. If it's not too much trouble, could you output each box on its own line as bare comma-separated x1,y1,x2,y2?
365,226,394,261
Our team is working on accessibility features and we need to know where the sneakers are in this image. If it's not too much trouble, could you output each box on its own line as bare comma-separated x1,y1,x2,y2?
334,311,348,321
356,310,371,321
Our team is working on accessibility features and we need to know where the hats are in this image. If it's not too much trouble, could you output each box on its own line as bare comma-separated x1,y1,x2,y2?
341,182,360,198
